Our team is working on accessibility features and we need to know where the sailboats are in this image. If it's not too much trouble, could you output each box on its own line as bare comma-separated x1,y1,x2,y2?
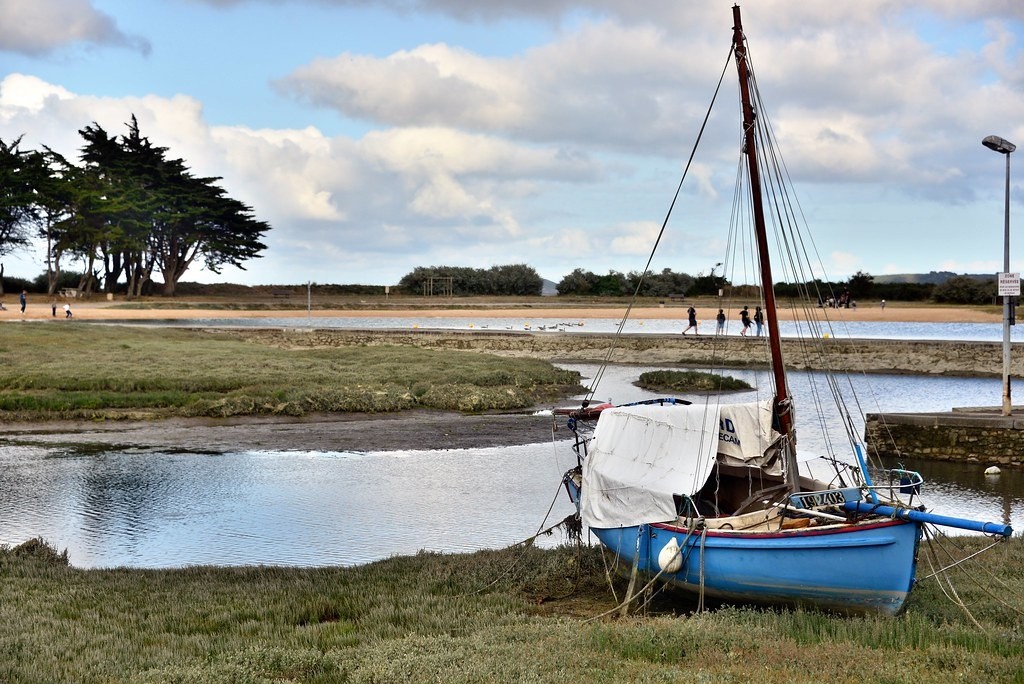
550,2,1017,619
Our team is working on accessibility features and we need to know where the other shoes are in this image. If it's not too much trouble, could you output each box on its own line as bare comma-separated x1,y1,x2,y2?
20,311,25,315
695,334,700,336
682,332,686,337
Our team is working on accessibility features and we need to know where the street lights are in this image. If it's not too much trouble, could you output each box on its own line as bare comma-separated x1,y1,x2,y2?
979,135,1018,417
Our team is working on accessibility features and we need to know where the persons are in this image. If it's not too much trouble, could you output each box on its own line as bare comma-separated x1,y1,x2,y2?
756,307,764,337
63,303,72,318
682,303,700,336
852,301,856,310
819,291,850,308
20,291,26,315
52,302,56,316
717,309,725,335
739,305,750,336
880,300,885,309
